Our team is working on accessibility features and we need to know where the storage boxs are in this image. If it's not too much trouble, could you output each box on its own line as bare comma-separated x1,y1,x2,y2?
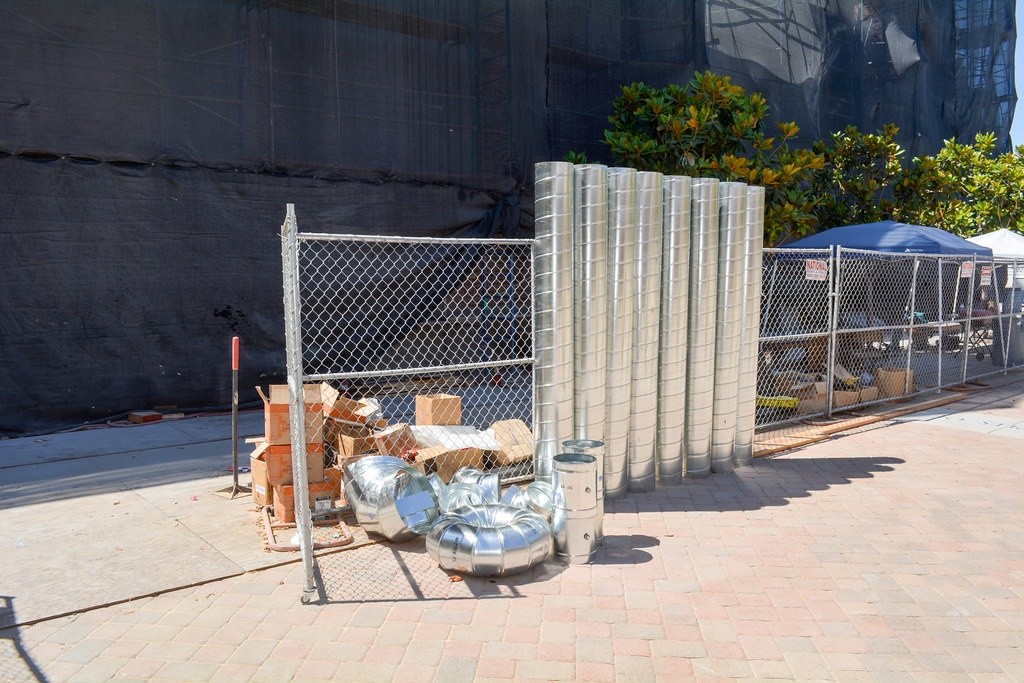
771,367,914,415
243,385,534,522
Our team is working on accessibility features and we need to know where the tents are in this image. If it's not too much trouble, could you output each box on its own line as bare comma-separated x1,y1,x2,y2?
762,220,1006,384
952,229,1024,375
